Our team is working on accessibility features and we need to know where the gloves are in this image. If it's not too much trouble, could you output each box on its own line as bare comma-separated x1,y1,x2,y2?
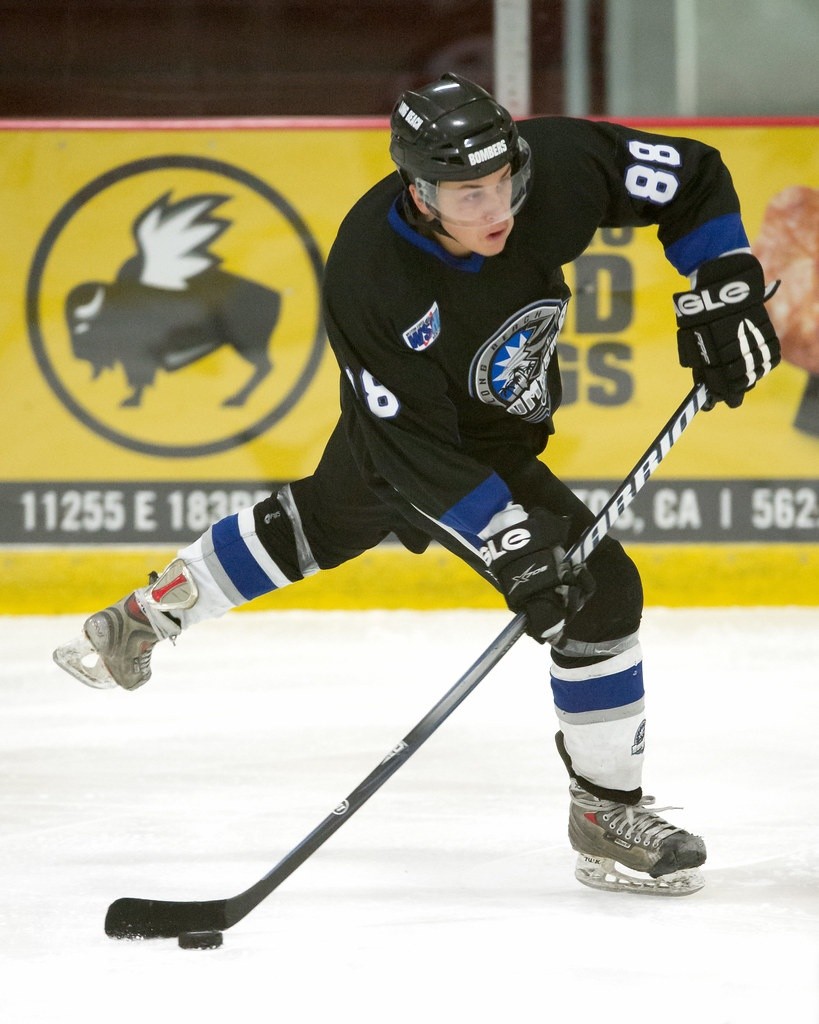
673,253,781,412
478,506,596,650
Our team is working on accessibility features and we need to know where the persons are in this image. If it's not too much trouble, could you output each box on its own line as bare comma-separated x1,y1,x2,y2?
54,73,781,896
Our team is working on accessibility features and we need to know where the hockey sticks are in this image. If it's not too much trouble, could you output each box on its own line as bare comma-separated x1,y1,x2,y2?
103,381,711,939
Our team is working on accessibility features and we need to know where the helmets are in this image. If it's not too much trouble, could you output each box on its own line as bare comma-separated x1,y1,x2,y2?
389,72,518,204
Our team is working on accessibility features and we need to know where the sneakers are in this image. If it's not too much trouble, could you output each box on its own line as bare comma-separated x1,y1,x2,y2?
555,730,707,894
52,558,198,691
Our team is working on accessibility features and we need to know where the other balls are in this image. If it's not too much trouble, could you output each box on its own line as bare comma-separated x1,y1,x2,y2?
177,929,224,950
